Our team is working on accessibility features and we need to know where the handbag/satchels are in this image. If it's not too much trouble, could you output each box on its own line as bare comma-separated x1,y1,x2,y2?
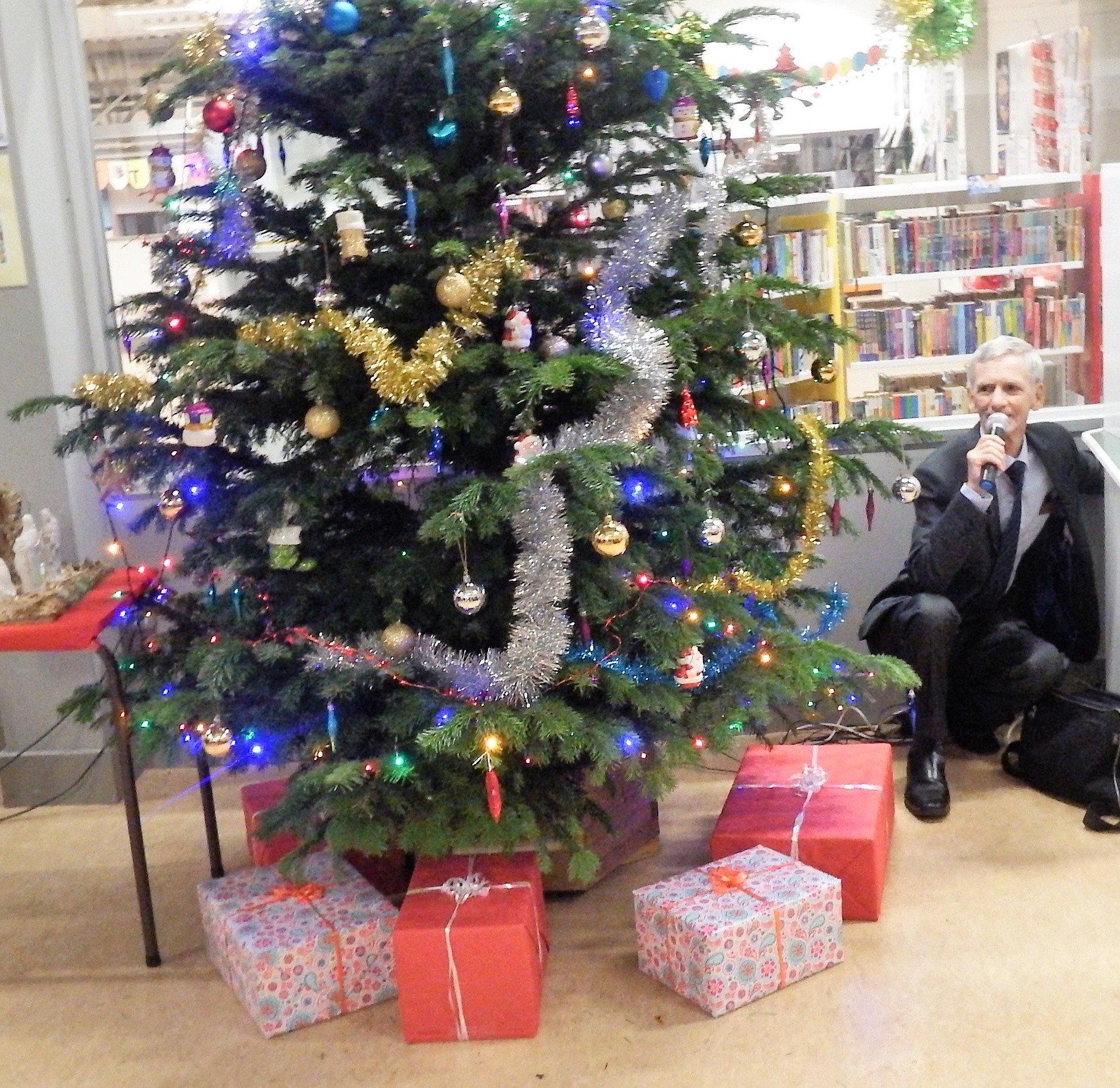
1014,674,1120,832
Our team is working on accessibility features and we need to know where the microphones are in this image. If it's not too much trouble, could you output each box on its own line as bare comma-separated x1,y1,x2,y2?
980,412,1011,492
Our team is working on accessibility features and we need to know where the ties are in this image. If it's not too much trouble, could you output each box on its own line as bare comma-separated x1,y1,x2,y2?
992,460,1026,592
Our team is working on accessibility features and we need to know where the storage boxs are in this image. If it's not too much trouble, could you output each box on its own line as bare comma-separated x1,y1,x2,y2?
392,850,549,1046
195,849,399,1039
240,778,411,895
632,844,843,1019
712,743,896,923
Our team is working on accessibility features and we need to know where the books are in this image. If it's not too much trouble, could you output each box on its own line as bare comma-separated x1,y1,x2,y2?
715,139,1088,423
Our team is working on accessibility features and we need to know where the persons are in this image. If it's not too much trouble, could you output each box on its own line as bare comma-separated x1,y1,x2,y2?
858,334,1104,818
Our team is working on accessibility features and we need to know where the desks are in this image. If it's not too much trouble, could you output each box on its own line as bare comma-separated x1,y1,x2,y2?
0,568,226,968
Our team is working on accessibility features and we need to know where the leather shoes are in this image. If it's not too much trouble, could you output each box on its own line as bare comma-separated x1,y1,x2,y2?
946,704,1000,755
904,749,951,823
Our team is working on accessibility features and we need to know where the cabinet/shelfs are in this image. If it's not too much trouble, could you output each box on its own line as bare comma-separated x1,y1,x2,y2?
505,126,1087,422
